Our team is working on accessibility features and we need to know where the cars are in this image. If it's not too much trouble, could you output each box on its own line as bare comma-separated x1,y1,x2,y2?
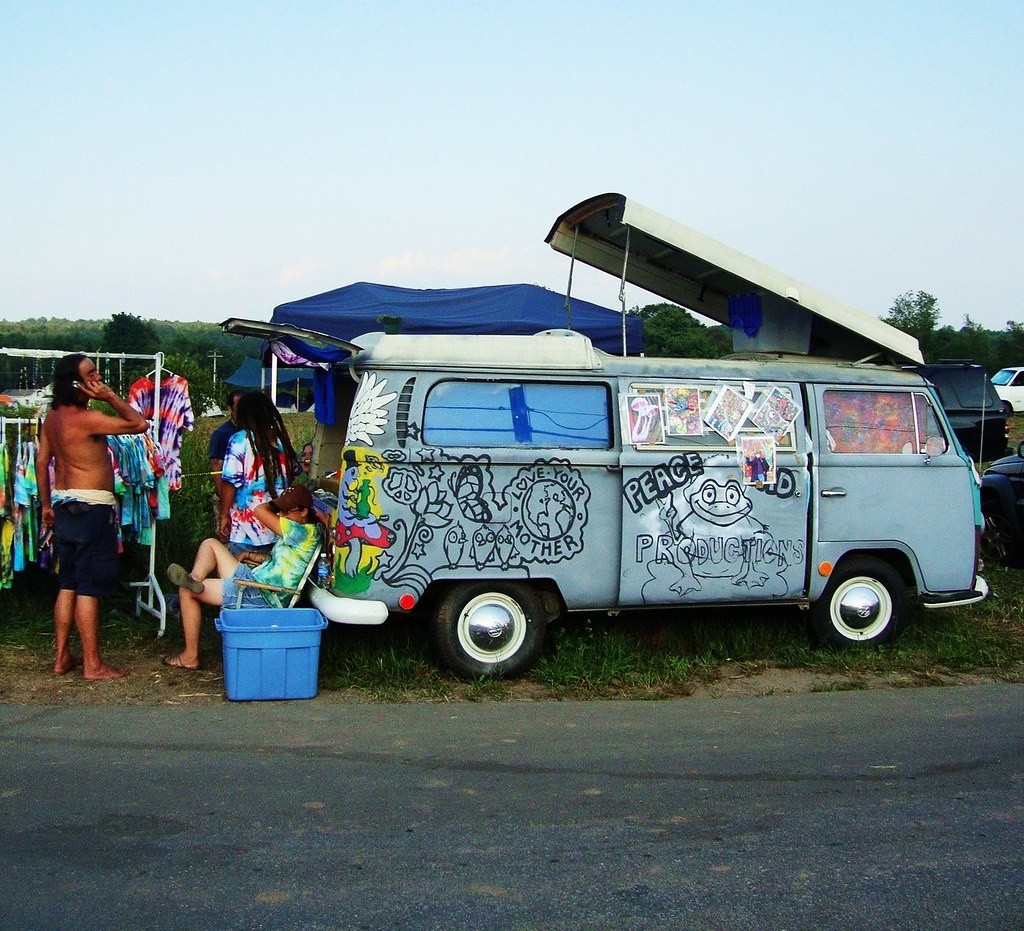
991,366,1024,418
979,441,1024,569
0,387,44,410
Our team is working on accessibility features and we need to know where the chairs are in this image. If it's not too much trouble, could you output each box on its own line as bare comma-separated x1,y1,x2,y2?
235,544,323,610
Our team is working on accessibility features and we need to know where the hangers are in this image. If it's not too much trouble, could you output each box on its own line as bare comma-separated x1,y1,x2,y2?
2,417,41,443
144,352,173,379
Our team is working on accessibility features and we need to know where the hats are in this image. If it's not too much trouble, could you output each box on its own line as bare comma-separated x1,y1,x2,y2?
269,483,314,512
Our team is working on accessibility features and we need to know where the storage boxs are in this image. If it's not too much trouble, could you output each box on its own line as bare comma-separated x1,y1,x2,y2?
213,608,328,701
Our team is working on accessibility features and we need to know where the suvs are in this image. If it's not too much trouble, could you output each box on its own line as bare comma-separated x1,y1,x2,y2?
897,356,1014,463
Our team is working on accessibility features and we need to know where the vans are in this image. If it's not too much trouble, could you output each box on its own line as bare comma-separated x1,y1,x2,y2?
214,193,990,686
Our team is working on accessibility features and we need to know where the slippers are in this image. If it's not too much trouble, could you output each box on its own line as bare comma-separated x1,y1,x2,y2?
167,564,204,593
162,655,202,670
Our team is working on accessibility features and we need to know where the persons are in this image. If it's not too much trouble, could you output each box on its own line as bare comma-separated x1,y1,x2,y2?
35,353,149,678
163,486,325,668
209,390,245,540
219,393,296,558
